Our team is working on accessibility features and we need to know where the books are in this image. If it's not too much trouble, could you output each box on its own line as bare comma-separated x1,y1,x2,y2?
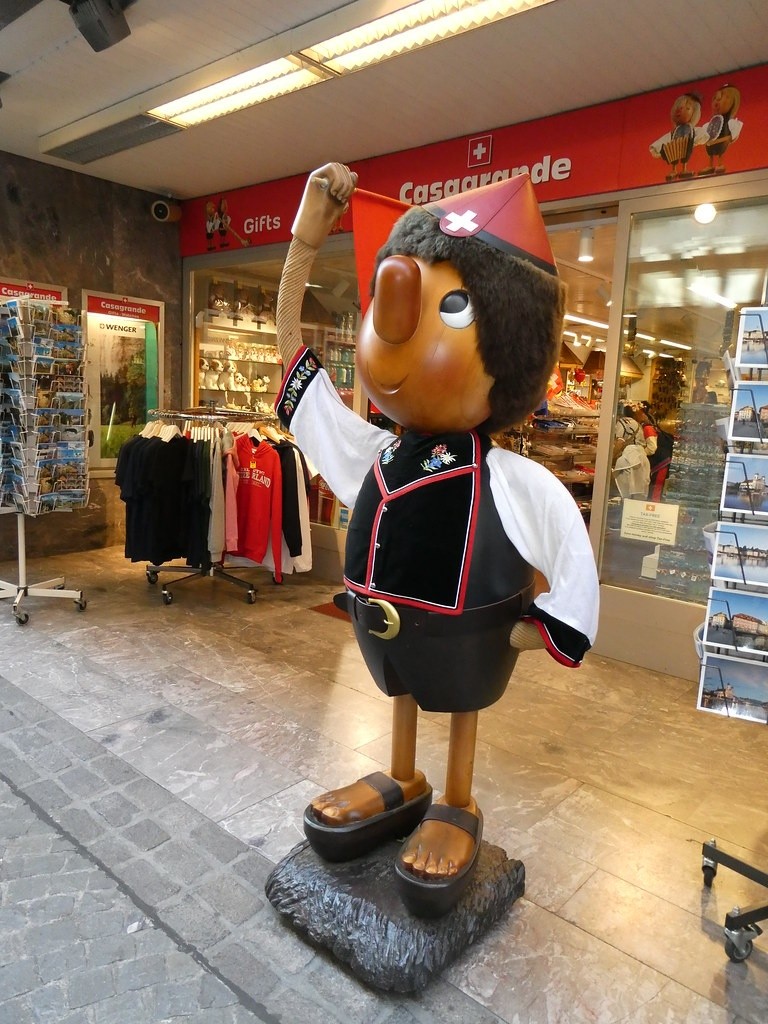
0,300,88,516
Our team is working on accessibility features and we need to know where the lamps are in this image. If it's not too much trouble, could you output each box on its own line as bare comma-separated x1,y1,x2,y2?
648,351,660,360
577,227,595,262
586,335,596,348
574,334,581,347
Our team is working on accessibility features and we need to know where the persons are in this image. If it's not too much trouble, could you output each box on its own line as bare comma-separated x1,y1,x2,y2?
273,164,601,916
609,399,672,531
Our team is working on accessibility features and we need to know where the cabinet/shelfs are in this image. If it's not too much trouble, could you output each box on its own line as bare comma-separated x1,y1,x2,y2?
197,315,283,414
524,405,622,517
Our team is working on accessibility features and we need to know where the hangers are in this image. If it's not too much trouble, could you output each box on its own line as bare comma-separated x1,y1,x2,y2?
136,409,294,448
